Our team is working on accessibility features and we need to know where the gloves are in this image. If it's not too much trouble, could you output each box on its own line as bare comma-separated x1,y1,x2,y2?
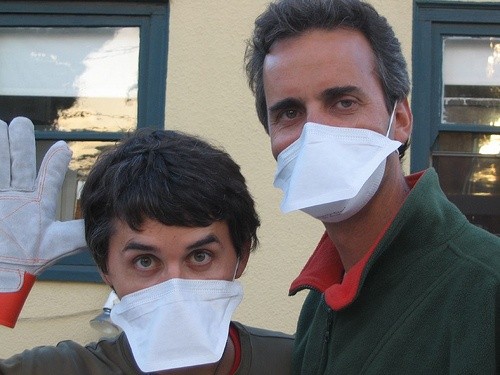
0,115,89,329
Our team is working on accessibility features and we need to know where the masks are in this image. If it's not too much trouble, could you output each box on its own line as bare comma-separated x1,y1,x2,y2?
273,99,403,223
110,255,244,373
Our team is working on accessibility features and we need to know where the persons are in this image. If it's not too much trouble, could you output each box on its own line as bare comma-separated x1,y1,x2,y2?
0,115,297,373
241,0,499,375
